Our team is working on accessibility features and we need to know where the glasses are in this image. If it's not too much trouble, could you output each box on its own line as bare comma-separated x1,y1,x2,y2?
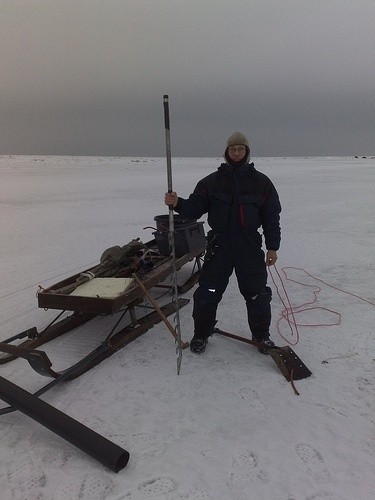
228,147,247,152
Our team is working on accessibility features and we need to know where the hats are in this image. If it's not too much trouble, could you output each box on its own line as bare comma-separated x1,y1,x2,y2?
223,132,251,166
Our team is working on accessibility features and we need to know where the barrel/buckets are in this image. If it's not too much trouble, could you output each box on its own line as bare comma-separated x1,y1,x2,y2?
154,214,197,231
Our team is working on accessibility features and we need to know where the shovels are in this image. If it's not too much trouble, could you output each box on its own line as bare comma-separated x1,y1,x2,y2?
212,327,312,382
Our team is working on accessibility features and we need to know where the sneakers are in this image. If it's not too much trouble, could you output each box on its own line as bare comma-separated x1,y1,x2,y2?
252,332,275,354
189,325,214,353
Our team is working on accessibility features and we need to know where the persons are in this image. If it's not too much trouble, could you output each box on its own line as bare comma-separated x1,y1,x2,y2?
164,131,281,354
